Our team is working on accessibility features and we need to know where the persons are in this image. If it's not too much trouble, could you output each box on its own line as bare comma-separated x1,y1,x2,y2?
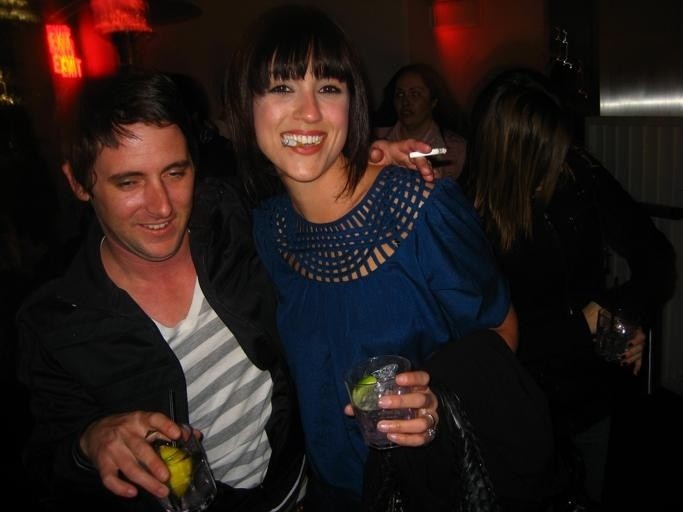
373,61,466,179
458,63,647,512
222,5,520,512
11,64,439,512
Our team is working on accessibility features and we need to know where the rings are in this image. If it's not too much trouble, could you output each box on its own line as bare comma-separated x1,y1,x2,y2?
424,412,437,436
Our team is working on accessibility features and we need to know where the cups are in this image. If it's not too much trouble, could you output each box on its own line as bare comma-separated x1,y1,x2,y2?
595,305,640,361
146,423,217,512
343,354,415,451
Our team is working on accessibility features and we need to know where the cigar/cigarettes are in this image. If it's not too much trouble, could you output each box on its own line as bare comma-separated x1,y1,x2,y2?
280,137,302,150
407,149,447,160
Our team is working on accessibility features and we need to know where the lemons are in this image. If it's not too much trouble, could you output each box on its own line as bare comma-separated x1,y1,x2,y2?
159,444,193,500
352,377,383,413
